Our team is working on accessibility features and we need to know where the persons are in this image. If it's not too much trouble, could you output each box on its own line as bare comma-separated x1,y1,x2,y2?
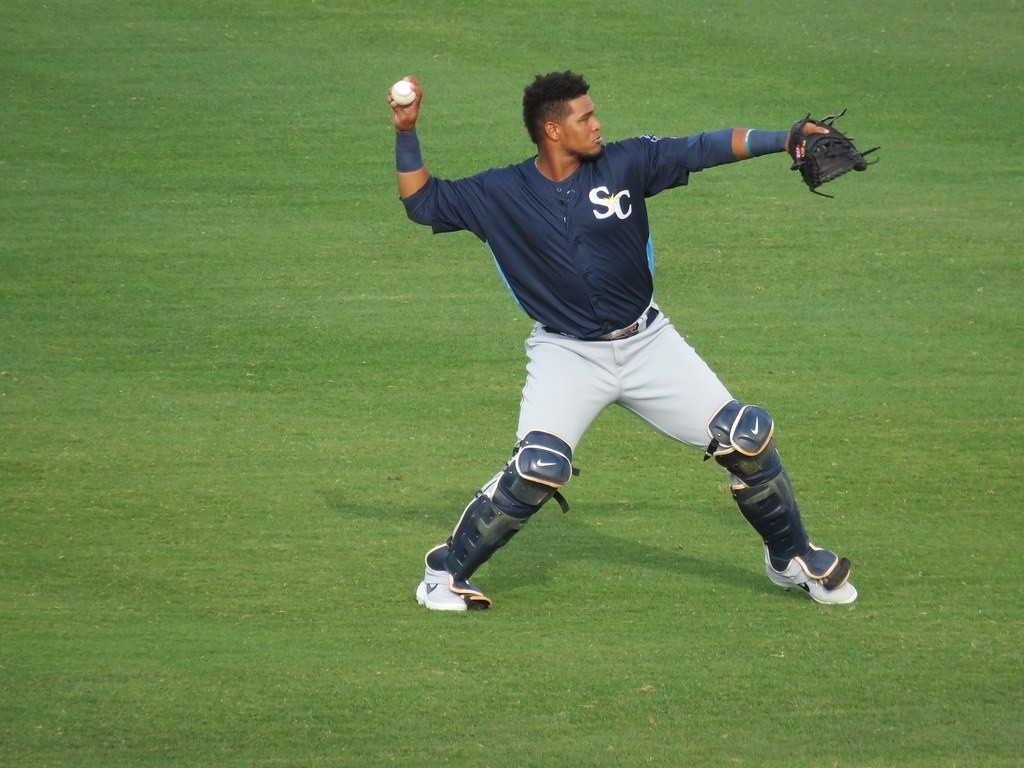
386,70,858,610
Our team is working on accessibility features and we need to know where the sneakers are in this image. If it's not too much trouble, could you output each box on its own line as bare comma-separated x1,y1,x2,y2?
762,540,858,604
415,566,494,612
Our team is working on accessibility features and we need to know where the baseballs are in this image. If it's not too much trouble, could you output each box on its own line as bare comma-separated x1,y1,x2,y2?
391,80,415,105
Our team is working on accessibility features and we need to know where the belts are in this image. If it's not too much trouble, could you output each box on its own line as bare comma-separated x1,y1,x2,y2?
540,307,659,342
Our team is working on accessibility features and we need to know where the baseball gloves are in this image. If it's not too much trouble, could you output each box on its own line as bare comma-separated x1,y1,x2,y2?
789,108,881,199
